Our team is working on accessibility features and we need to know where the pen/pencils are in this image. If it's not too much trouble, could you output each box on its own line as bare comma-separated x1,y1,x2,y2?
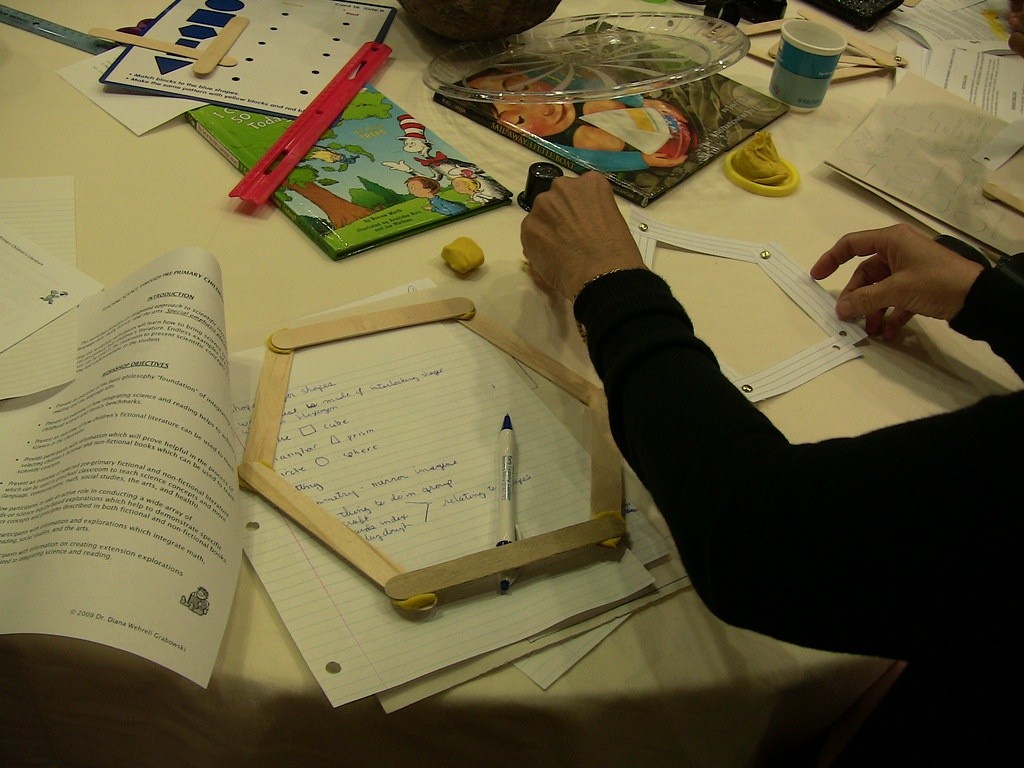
493,411,520,591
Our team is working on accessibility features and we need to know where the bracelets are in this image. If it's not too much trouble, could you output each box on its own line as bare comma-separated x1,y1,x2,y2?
572,268,620,343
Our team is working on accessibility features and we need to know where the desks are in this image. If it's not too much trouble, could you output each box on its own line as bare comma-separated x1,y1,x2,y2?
0,0,1024,767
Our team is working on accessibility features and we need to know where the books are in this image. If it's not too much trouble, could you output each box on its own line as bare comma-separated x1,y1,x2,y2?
434,20,790,207
187,78,513,261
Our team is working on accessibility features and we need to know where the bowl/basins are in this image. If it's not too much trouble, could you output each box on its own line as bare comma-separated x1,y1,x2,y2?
397,0,561,42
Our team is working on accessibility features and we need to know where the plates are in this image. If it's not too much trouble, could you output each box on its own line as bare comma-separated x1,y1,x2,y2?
421,11,751,104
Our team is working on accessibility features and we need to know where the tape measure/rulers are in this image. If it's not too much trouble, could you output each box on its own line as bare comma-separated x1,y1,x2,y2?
0,3,119,56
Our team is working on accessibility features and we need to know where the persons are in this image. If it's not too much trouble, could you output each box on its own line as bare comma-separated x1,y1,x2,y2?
520,171,1024,768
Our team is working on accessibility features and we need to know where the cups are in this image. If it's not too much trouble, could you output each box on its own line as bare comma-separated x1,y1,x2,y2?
769,20,848,113
